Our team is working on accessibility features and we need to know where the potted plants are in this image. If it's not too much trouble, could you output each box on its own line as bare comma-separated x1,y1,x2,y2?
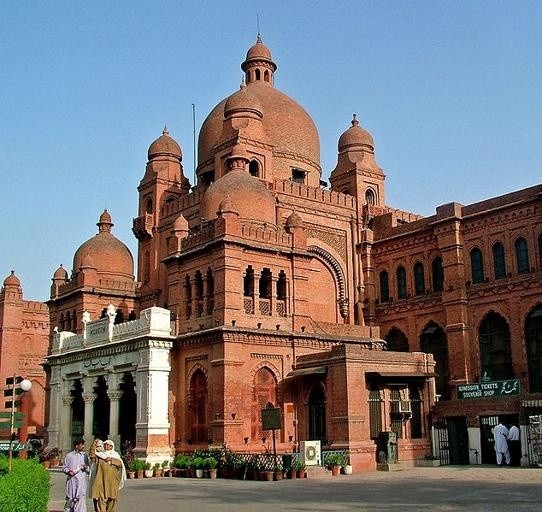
40,446,62,469
122,439,306,481
324,449,352,475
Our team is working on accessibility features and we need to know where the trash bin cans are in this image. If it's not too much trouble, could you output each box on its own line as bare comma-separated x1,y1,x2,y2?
378,432,397,464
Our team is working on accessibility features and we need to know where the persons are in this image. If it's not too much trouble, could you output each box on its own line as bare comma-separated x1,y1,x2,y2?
62,438,127,512
493,418,520,466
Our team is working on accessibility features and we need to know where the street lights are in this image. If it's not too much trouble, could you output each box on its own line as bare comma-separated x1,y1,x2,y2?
9,374,32,472
